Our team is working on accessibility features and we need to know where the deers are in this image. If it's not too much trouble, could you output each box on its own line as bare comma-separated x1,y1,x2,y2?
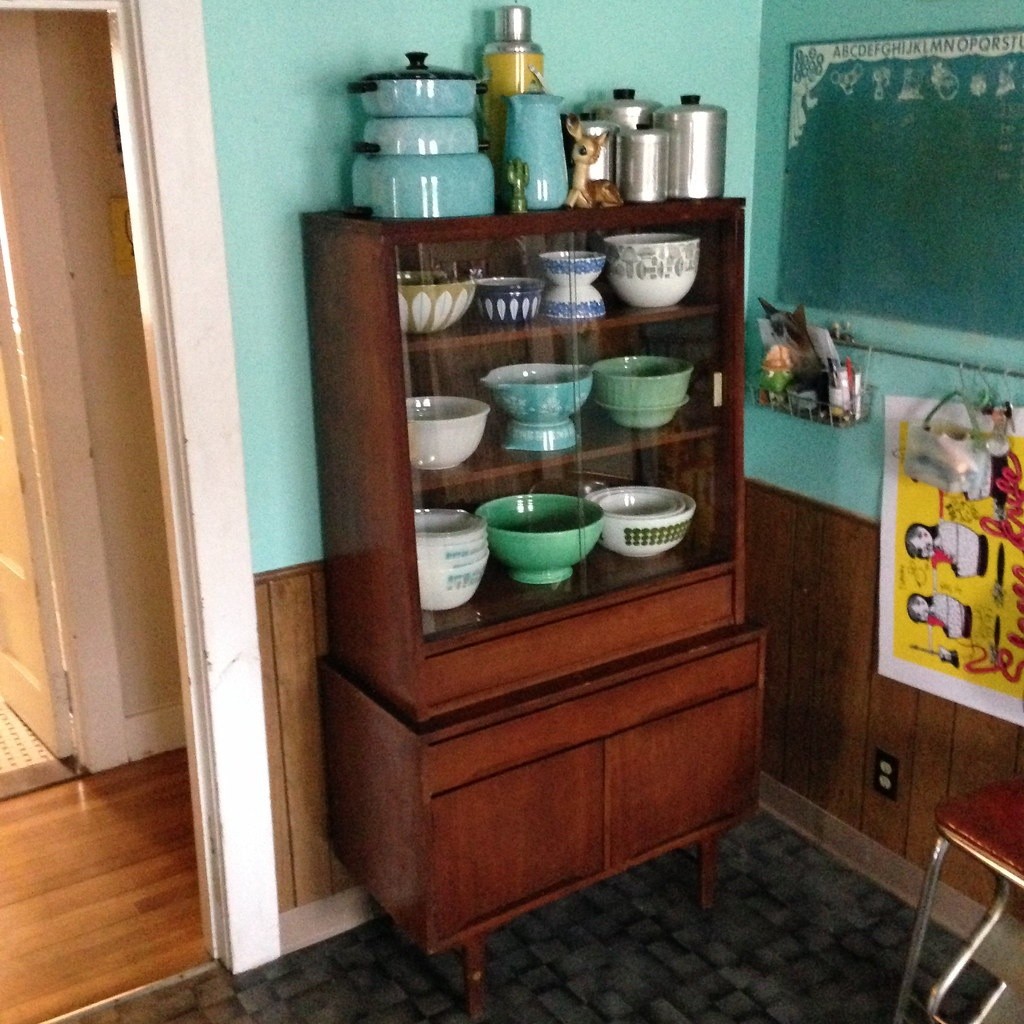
562,113,624,209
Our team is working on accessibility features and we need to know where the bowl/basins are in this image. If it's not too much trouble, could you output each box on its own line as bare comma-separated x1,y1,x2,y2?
538,251,606,287
474,276,545,325
594,394,689,428
539,286,606,318
502,418,578,450
405,397,491,470
590,489,687,520
585,485,697,556
480,363,593,422
416,533,488,561
603,232,701,308
416,548,488,611
414,509,487,532
599,491,678,515
475,494,605,583
417,540,491,569
590,356,695,408
396,270,477,334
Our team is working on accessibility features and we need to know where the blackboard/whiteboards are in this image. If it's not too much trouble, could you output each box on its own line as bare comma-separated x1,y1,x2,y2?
774,26,1024,343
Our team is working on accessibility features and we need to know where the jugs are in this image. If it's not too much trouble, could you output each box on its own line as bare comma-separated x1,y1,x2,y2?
476,5,543,213
502,91,568,209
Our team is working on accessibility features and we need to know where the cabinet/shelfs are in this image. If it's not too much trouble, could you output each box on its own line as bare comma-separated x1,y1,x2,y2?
299,196,772,1023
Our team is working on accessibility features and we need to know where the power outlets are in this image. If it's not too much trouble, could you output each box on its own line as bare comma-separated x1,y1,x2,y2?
873,746,899,802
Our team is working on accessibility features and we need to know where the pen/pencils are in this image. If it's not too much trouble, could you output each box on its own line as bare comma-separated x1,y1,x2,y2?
847,355,861,420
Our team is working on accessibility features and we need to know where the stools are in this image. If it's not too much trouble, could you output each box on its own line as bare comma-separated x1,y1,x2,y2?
891,776,1024,1024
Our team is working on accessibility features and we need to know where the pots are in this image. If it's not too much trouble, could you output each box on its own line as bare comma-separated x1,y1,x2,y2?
345,51,488,116
347,154,503,218
352,117,491,153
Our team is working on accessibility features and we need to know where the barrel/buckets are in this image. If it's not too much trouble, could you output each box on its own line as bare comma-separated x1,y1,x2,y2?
651,94,727,199
616,121,669,203
590,89,659,130
